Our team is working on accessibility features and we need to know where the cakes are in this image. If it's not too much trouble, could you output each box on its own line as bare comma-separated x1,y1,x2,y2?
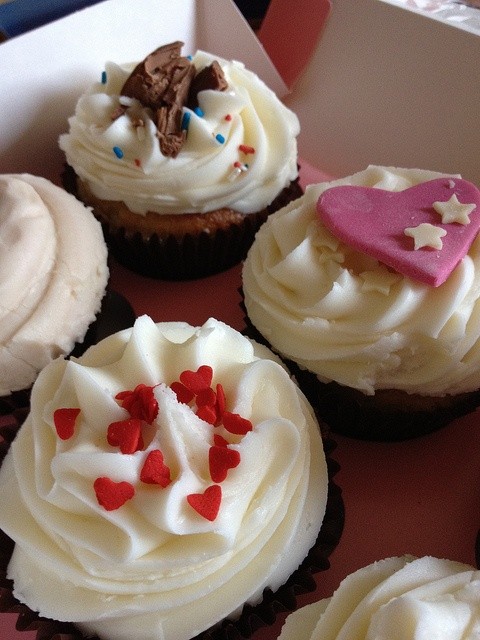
2,311,346,635
1,172,110,396
279,552,478,639
241,163,480,444
58,41,302,284
